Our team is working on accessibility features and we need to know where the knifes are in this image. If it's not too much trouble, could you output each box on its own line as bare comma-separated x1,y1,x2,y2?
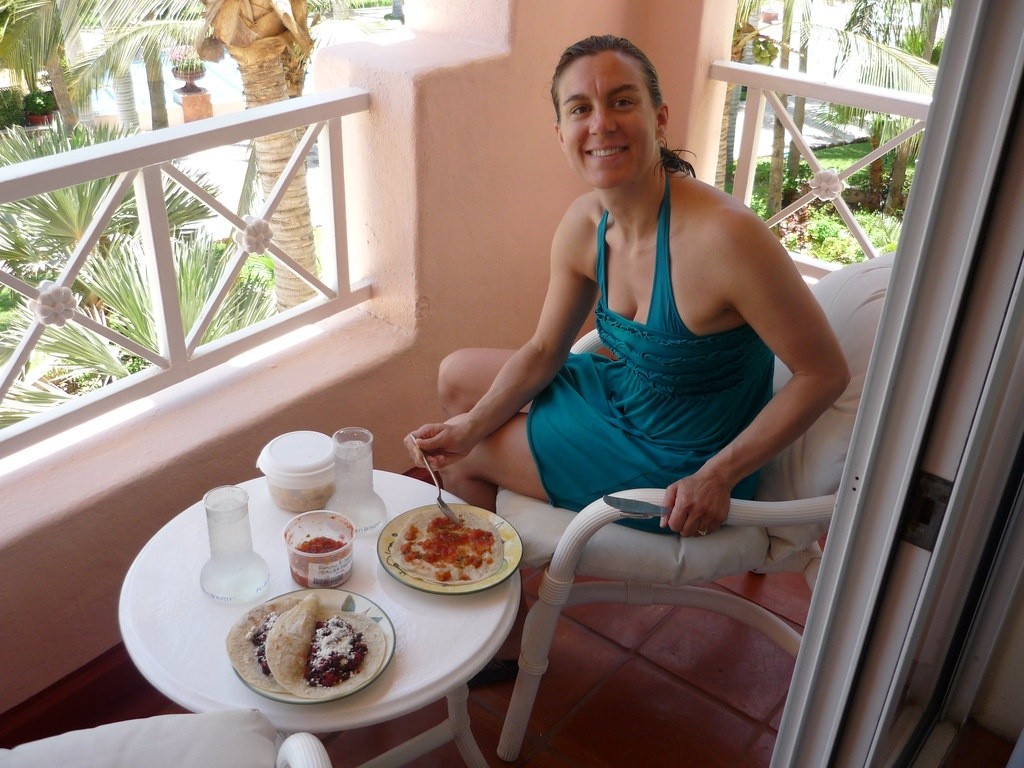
603,495,672,514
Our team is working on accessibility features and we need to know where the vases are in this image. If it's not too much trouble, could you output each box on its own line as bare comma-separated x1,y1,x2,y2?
174,69,207,94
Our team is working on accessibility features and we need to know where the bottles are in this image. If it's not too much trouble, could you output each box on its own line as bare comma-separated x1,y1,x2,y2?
324,426,386,538
199,484,271,606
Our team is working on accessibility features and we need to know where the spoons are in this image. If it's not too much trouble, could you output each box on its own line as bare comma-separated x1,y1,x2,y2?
410,435,461,528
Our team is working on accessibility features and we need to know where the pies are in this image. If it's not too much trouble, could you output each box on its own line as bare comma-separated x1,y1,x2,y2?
225,593,385,700
391,510,504,586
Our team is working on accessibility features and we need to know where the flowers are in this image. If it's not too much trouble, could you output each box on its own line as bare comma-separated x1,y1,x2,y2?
171,45,202,71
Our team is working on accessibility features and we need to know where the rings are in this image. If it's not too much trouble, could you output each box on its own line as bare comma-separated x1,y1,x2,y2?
697,530,708,536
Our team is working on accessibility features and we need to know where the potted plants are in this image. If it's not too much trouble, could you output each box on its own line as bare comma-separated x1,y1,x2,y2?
23,91,56,125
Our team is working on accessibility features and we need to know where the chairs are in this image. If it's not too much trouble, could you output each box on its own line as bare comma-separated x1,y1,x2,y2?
495,251,898,762
0,707,333,768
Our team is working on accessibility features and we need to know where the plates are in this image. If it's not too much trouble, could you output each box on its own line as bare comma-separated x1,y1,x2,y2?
232,587,396,705
377,503,524,595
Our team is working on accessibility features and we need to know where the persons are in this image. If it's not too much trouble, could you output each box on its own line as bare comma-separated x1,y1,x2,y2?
402,35,853,690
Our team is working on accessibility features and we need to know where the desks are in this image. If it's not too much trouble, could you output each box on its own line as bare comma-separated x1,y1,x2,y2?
118,467,521,768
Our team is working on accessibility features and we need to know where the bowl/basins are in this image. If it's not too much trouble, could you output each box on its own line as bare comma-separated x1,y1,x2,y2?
282,510,356,589
256,431,334,513
